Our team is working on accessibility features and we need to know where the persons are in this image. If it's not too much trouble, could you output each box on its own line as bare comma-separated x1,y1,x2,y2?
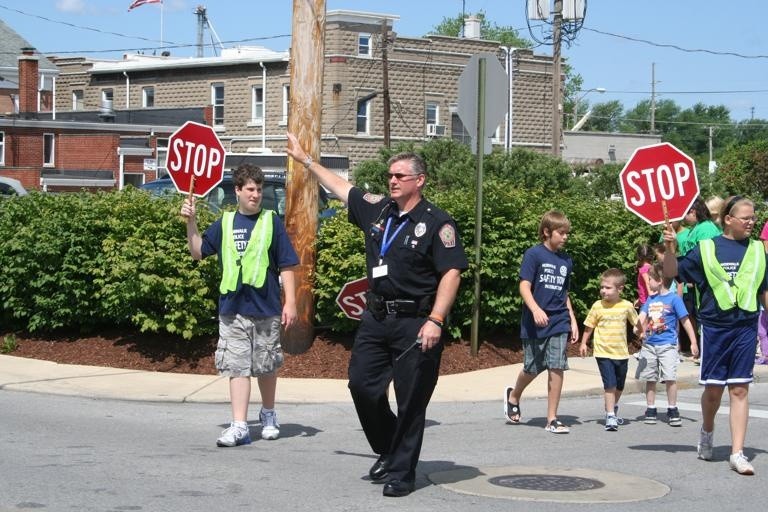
504,211,579,434
662,194,767,475
282,131,462,496
632,194,767,429
180,163,300,448
580,267,644,430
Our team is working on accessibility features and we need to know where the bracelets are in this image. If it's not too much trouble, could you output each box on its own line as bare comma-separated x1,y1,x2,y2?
427,318,444,330
428,313,445,322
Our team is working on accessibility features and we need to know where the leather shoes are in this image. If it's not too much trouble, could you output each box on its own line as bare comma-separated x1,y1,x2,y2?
370,456,415,497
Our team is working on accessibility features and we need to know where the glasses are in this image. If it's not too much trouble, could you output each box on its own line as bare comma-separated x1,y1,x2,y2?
388,173,412,178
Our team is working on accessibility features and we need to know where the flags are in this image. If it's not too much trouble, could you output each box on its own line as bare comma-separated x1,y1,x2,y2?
126,0,162,12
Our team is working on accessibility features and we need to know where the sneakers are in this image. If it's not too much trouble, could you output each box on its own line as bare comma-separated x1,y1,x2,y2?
729,451,754,475
259,408,280,440
216,426,251,447
698,427,712,459
665,407,681,426
605,414,618,431
644,408,657,424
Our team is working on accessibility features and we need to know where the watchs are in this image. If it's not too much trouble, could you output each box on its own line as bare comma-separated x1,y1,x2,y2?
301,153,313,168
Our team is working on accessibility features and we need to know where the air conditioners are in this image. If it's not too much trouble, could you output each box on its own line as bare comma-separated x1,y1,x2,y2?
426,123,448,137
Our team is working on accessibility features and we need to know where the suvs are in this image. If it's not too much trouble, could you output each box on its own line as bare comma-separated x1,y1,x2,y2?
127,170,343,240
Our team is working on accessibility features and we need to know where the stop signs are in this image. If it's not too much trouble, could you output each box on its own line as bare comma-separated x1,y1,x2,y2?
615,141,700,229
334,277,369,322
163,119,227,200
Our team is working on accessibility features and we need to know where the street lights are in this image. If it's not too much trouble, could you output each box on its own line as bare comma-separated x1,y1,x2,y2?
572,87,606,109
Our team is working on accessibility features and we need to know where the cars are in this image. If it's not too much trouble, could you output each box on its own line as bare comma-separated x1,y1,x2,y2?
0,176,29,199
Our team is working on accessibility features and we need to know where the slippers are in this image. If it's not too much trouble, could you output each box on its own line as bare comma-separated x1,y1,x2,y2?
504,386,520,423
545,419,570,433
